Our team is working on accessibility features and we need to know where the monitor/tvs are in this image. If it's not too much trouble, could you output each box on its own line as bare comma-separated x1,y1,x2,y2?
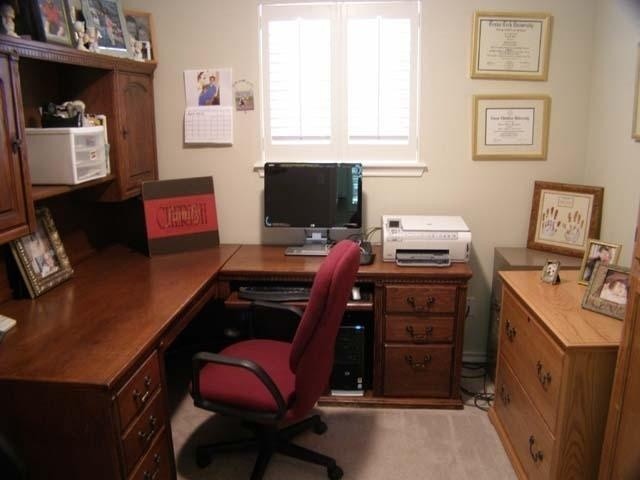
263,161,363,257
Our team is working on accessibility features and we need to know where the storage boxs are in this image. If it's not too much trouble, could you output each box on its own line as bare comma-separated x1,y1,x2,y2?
26,126,108,185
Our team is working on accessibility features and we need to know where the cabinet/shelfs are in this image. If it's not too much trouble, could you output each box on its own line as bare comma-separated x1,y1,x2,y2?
17,41,116,201
484,246,592,384
112,51,159,201
0,35,37,245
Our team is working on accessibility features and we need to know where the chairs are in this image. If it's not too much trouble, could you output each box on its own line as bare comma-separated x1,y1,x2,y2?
190,240,361,480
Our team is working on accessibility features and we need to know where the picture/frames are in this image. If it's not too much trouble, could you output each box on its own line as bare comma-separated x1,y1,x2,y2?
582,261,631,320
470,93,552,162
527,180,605,259
9,207,74,300
469,9,552,82
34,0,76,49
82,0,134,58
577,239,622,289
631,42,640,140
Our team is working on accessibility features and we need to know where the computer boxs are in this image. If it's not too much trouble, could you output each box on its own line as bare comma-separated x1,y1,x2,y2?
330,324,366,398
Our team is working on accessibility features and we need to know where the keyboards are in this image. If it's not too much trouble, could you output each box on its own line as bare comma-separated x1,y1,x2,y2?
237,283,314,303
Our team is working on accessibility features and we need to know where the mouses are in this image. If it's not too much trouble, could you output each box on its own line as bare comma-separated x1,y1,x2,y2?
349,287,365,301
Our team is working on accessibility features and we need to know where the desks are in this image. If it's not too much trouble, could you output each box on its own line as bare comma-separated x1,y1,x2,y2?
219,271,473,409
2,178,242,480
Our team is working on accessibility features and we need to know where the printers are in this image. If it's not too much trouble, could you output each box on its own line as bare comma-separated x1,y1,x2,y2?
381,214,472,268
